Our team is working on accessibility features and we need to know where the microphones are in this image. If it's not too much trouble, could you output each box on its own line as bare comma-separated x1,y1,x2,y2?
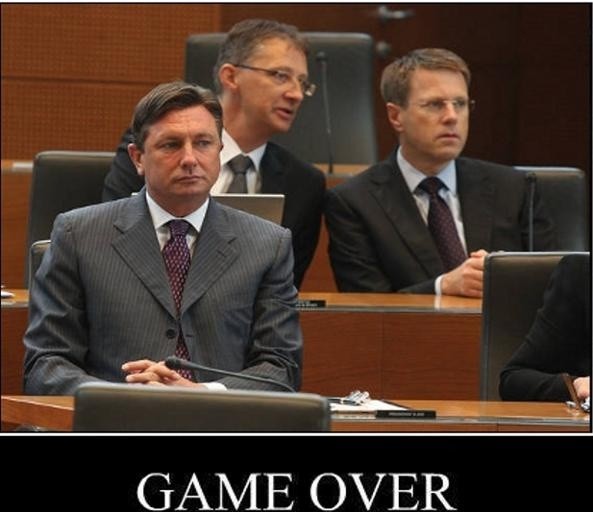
525,171,538,252
316,50,336,175
164,354,301,394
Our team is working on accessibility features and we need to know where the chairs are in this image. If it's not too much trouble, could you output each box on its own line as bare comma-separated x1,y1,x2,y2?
70,376,336,432
24,144,131,292
27,231,56,286
477,247,580,403
511,163,591,253
182,24,379,165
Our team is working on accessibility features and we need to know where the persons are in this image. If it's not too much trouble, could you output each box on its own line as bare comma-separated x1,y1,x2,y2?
497,255,590,403
323,47,558,301
101,19,326,294
20,78,305,396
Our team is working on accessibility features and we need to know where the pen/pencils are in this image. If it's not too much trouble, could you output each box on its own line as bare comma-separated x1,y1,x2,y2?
328,397,361,406
565,400,590,413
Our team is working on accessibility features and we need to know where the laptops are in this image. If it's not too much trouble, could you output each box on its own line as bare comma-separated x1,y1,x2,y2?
132,192,286,228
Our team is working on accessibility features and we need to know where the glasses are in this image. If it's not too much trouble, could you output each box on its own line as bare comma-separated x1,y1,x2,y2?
236,65,316,97
420,101,477,115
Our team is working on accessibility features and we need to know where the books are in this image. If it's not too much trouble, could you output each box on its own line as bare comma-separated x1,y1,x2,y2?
329,395,411,419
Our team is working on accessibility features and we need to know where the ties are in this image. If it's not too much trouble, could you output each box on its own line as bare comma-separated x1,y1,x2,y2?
161,219,195,383
226,155,252,193
418,178,466,270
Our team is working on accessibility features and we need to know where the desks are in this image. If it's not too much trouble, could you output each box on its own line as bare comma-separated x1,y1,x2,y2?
0,149,376,293
1,382,592,439
1,278,499,407
